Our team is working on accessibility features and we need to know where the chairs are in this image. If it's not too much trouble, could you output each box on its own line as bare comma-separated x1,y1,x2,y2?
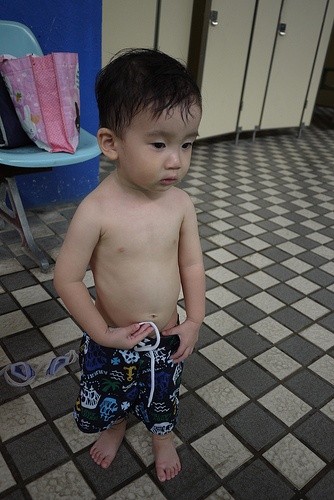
0,20,102,274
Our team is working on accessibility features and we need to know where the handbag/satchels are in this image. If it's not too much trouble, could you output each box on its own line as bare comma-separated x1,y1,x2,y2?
0,51,81,153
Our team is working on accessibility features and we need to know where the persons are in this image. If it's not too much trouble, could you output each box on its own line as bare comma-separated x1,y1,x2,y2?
53,48,206,482
